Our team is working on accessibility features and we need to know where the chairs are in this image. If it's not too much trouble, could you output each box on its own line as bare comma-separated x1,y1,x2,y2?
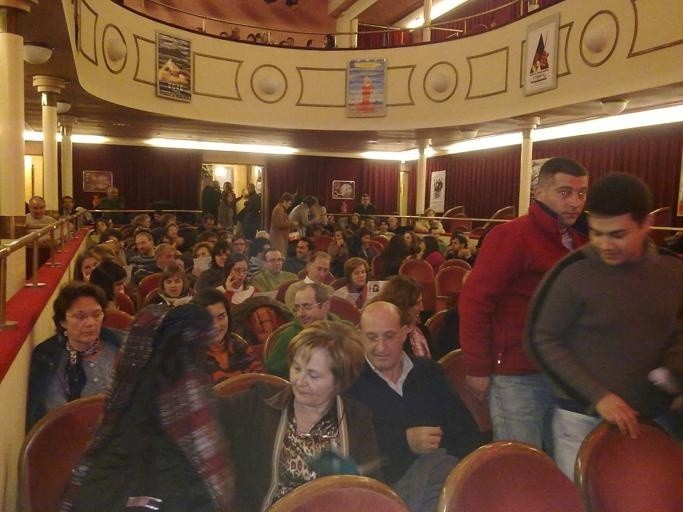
261,473,409,511
437,441,583,510
69,197,670,431
18,395,118,511
574,417,682,512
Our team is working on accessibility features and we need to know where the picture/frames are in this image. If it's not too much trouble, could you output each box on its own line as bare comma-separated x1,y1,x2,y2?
331,179,356,201
344,56,387,120
154,30,192,102
430,168,446,214
80,169,114,195
522,12,560,98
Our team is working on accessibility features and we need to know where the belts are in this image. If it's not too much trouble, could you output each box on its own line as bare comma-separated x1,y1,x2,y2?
555,399,665,421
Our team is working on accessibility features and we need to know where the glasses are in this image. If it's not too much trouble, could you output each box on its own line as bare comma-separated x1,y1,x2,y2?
294,301,321,314
292,402,346,442
64,310,104,321
366,324,403,342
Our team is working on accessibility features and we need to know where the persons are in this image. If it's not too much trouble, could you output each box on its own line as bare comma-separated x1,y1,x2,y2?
459,155,587,452
344,306,483,511
28,282,124,428
526,174,682,479
62,305,240,512
225,319,384,511
27,171,483,378
218,26,338,50
